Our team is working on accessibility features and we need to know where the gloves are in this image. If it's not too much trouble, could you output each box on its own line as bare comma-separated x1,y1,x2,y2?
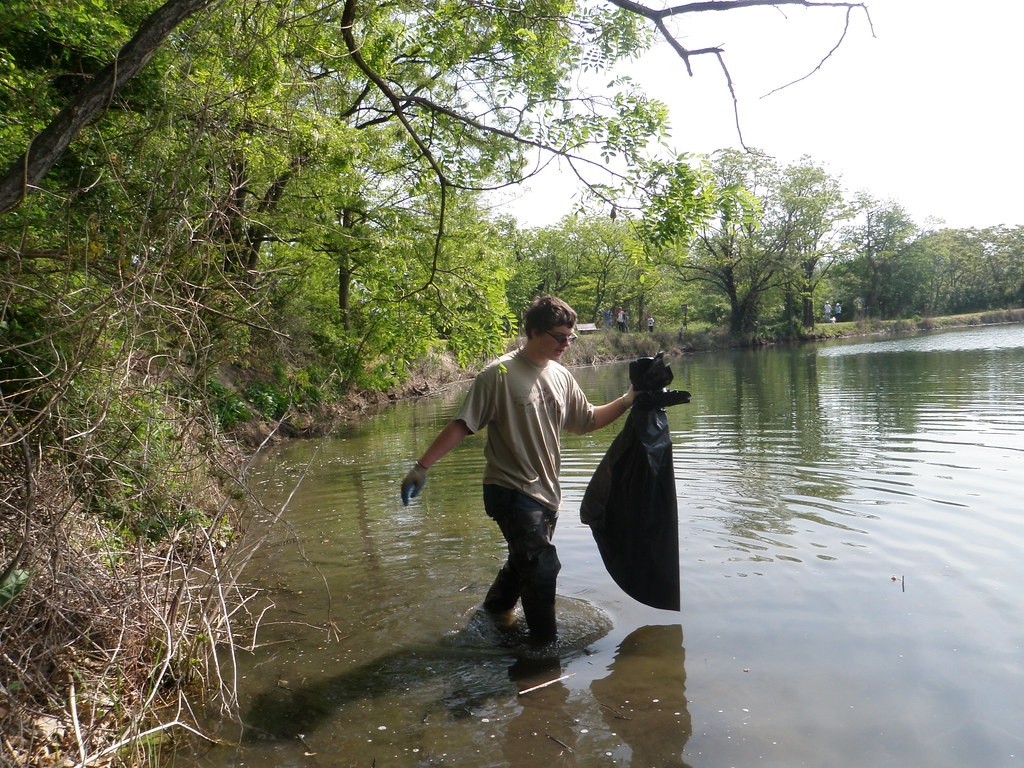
622,383,643,409
401,461,429,506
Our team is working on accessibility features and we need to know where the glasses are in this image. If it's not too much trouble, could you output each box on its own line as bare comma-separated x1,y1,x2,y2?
543,329,578,347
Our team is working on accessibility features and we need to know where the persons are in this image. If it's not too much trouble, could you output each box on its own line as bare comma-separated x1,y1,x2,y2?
647,315,655,332
616,306,630,332
399,294,633,644
835,303,841,322
603,306,613,328
823,301,832,319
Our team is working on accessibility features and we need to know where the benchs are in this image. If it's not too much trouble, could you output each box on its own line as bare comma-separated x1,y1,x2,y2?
575,323,601,334
